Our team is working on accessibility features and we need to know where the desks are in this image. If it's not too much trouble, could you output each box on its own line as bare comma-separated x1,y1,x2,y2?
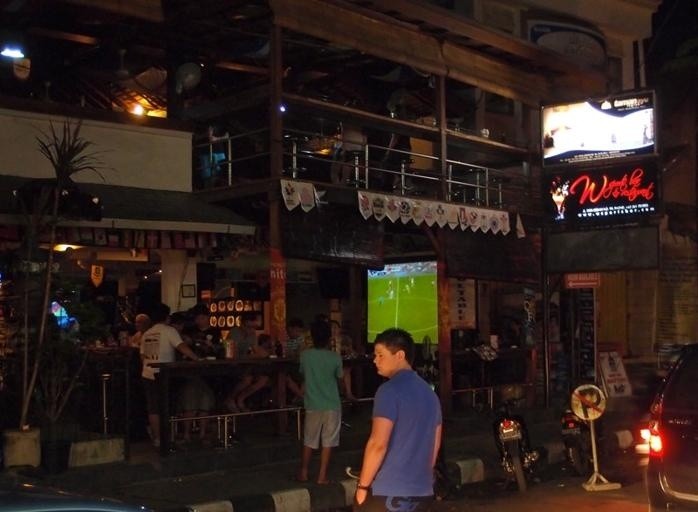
147,351,371,448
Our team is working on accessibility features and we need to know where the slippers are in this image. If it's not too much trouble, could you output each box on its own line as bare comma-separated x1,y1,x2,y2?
318,479,338,486
289,473,309,482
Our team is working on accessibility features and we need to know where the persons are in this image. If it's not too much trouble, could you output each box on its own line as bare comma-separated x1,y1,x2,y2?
128,303,358,449
330,96,368,184
352,328,443,512
184,97,226,189
490,315,537,400
296,320,346,489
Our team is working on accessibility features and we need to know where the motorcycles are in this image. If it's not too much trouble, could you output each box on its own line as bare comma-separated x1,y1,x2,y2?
489,386,590,495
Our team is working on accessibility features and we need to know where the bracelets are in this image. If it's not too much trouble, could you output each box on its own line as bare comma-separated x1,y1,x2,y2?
356,483,368,490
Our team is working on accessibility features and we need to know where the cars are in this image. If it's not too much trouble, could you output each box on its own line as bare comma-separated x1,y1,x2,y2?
647,344,698,511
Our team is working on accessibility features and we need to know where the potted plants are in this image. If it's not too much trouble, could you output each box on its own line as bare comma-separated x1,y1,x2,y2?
2,112,118,469
